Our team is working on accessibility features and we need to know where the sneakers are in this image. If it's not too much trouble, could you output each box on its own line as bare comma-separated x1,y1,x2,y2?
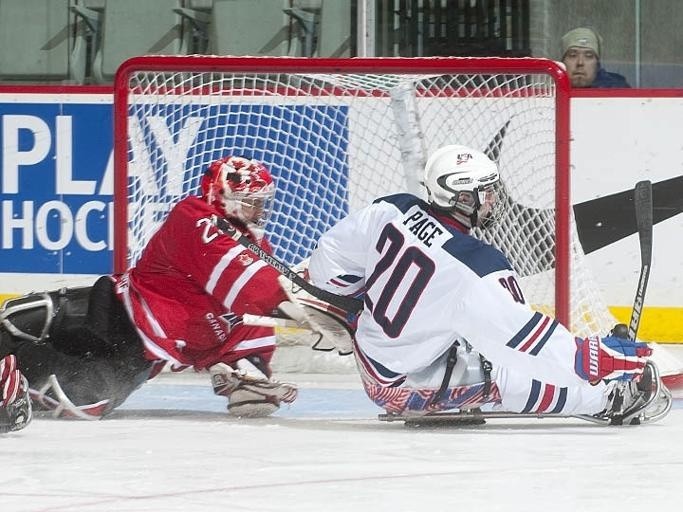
604,323,630,342
0,353,28,404
607,362,654,420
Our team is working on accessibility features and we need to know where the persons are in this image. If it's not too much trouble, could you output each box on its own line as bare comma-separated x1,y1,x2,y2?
560,28,632,89
272,143,672,426
0,154,317,432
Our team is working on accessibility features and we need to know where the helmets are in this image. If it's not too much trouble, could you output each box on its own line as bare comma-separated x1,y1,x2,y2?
199,156,273,212
424,145,499,209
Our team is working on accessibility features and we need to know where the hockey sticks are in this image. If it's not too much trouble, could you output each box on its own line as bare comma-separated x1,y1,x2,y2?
208,213,364,313
611,180,653,426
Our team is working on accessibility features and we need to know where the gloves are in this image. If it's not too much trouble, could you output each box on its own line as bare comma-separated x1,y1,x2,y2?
209,355,297,417
574,335,655,385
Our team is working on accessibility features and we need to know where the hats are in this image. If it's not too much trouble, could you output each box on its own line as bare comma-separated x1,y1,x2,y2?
561,27,602,63
